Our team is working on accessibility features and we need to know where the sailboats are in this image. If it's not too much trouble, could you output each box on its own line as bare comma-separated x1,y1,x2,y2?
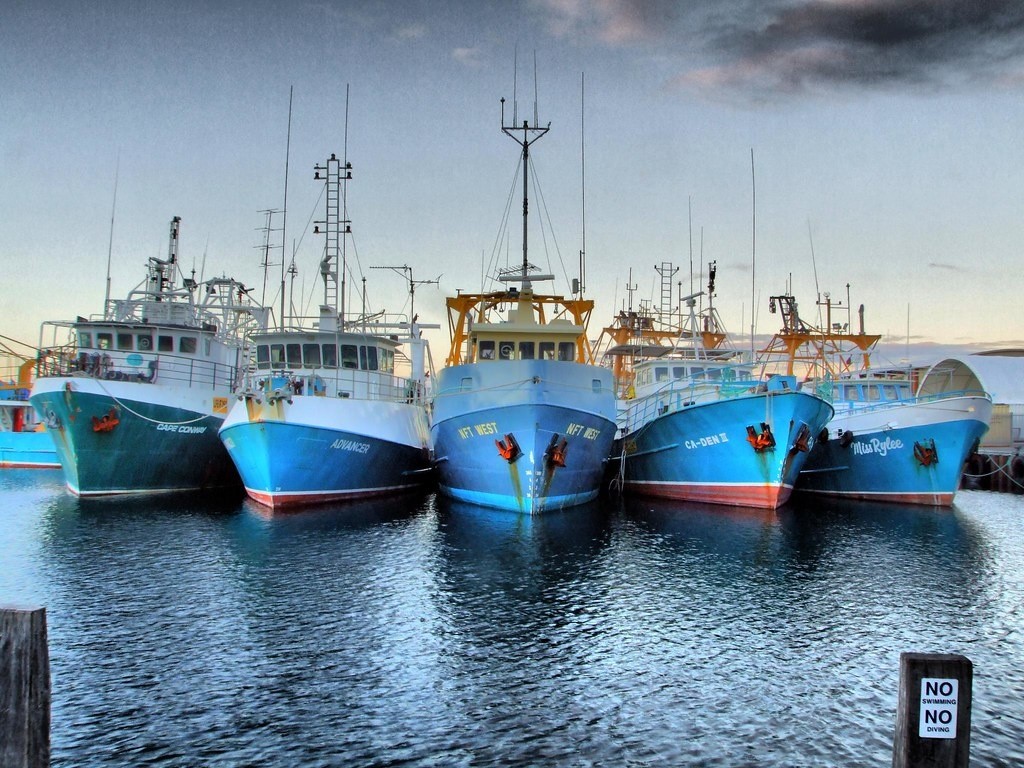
0,40,995,517
